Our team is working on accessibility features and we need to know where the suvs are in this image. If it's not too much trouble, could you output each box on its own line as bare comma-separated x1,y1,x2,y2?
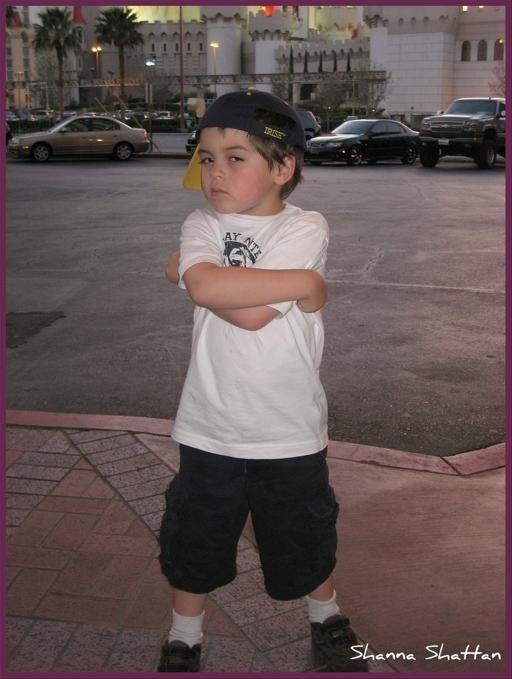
419,95,506,171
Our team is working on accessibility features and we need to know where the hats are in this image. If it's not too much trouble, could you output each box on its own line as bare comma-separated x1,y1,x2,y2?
182,89,306,192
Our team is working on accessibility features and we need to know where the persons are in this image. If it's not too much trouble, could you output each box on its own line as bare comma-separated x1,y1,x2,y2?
157,89,371,672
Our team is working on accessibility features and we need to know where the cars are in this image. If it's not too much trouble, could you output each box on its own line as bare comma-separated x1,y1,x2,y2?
7,114,152,161
185,111,324,168
303,118,417,167
4,107,188,132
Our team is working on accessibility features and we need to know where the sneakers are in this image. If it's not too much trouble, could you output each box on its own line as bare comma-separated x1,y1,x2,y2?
157,638,202,672
311,611,369,671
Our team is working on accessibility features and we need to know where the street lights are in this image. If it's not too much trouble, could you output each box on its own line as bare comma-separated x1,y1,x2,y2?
144,59,156,102
209,41,221,102
91,46,101,78
